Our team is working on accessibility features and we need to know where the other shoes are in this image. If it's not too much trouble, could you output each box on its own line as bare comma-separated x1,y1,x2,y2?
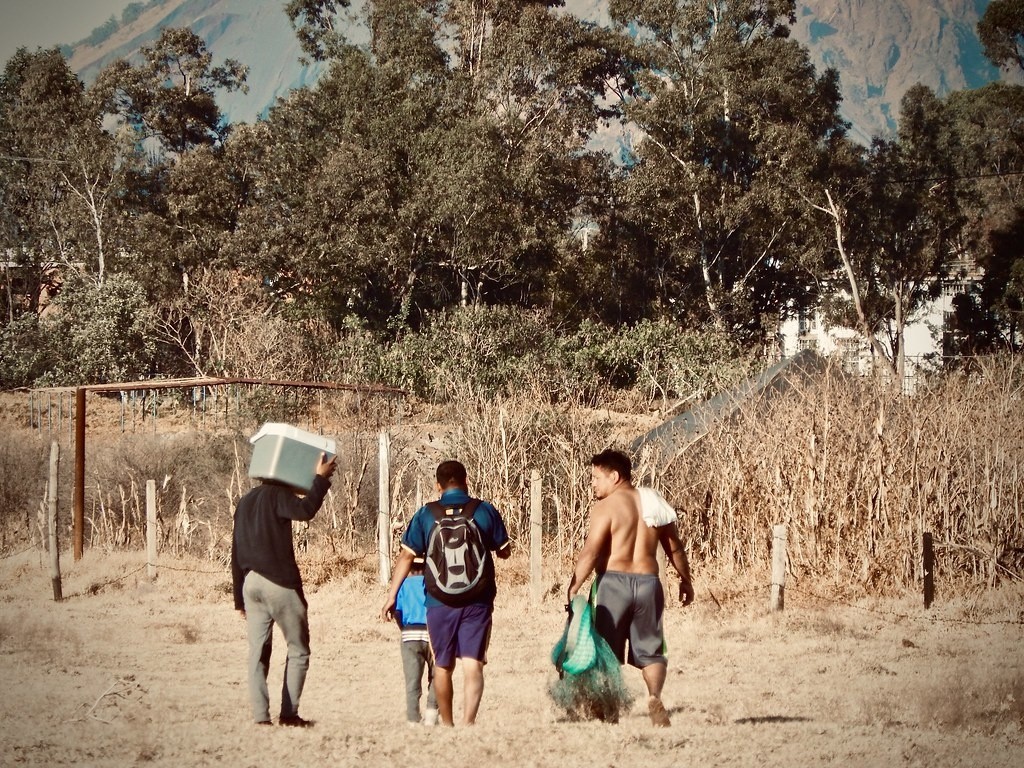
648,695,670,726
279,715,311,727
424,708,439,727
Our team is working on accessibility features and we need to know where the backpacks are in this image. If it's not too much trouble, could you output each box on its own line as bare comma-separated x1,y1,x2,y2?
425,500,495,607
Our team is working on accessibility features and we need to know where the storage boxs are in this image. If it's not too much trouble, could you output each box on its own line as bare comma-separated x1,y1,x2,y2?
247,421,336,492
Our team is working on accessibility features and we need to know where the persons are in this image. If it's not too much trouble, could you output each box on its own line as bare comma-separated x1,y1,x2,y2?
231,450,338,728
567,449,694,726
380,460,516,728
